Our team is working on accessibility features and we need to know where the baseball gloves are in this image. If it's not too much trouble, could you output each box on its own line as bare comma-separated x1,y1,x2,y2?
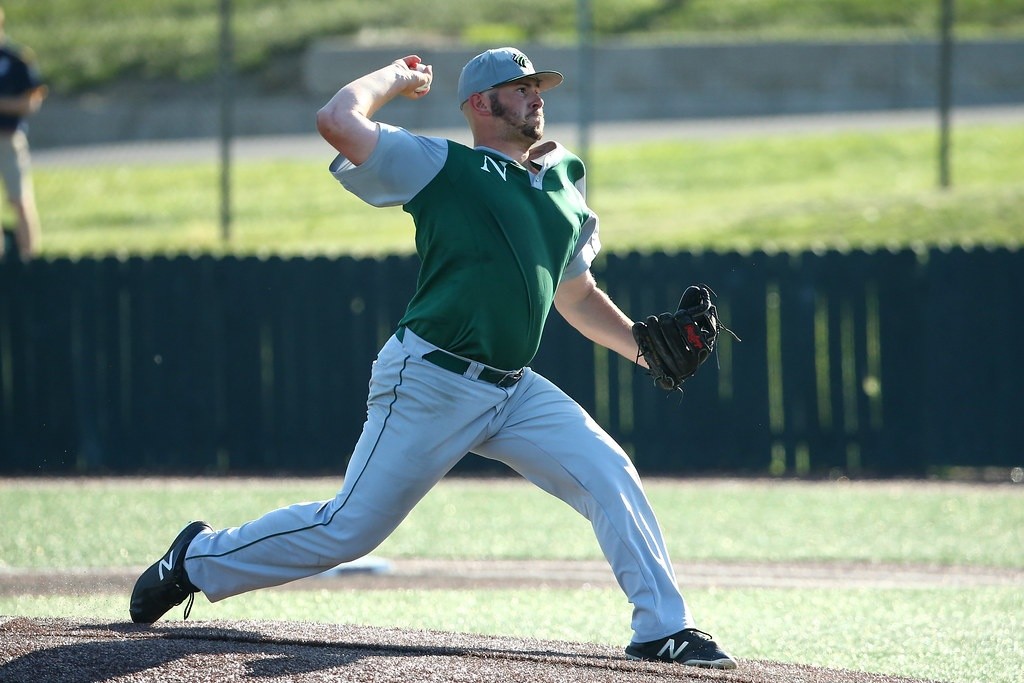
631,286,718,390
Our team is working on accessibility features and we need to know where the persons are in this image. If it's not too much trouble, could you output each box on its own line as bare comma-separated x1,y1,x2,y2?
130,47,739,671
0,9,49,260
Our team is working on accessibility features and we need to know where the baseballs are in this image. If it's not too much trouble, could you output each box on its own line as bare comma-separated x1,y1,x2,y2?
410,64,432,92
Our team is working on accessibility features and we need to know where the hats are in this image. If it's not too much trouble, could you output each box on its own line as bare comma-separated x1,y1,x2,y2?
458,48,564,111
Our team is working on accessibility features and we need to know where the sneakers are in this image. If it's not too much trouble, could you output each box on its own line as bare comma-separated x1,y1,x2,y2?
625,626,738,669
129,520,215,625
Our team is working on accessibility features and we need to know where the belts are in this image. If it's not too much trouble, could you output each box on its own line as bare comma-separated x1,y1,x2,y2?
395,325,525,387
